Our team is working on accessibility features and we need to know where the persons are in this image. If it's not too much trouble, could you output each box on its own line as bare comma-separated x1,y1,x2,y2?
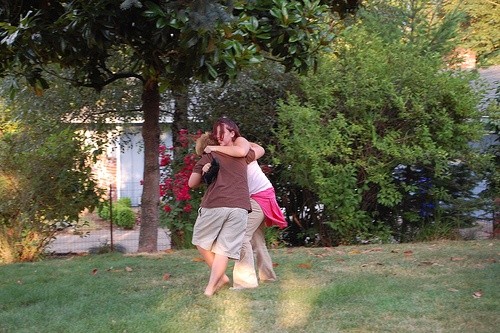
204,116,287,282
188,132,265,297
